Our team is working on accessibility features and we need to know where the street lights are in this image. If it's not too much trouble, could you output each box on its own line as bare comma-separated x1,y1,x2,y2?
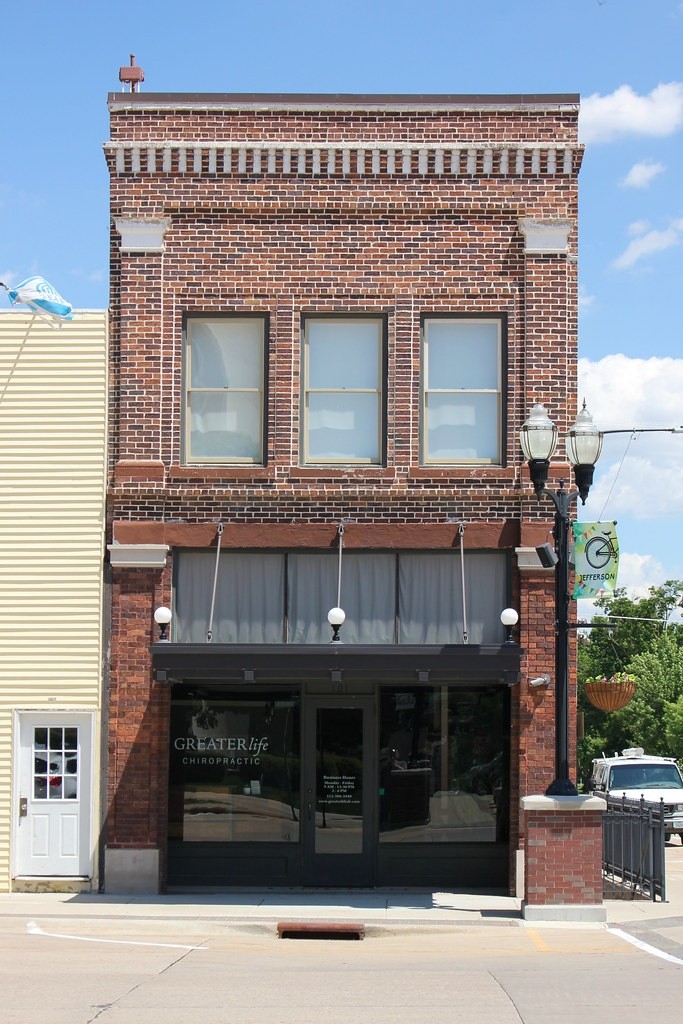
520,399,606,796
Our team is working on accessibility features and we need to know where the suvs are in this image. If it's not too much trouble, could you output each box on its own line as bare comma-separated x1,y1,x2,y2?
591,747,683,845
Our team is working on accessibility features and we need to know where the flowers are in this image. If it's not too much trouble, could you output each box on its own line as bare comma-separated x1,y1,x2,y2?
586,672,640,685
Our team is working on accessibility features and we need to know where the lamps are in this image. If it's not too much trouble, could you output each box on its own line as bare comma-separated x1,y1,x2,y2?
244,671,254,681
154,607,173,644
529,673,552,688
328,608,345,646
500,608,519,647
331,671,341,682
157,671,167,681
418,672,429,682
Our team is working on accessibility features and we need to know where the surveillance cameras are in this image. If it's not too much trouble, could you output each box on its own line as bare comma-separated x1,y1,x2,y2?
529,677,545,687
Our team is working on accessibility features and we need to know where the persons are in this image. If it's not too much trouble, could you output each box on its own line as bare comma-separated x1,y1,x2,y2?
389,748,407,771
